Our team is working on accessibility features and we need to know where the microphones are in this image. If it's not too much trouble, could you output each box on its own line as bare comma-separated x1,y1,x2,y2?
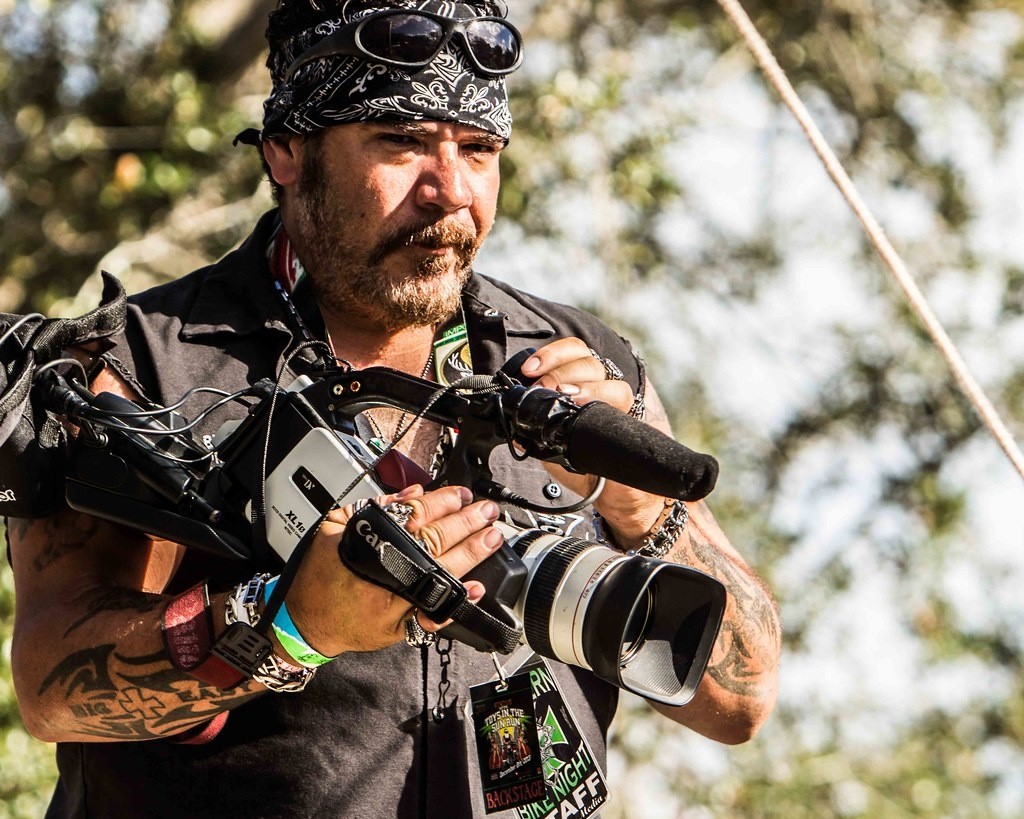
505,385,719,501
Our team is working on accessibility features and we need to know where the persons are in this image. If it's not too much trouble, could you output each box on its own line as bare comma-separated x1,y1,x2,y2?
0,0,782,819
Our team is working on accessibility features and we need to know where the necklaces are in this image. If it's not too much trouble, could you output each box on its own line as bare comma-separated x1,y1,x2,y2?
276,280,450,480
364,348,434,453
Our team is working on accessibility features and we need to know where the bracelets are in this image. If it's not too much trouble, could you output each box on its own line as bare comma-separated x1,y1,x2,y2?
592,492,688,559
225,571,338,694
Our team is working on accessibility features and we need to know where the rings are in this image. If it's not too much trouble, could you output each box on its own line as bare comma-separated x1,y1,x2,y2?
355,499,362,514
628,394,645,420
600,358,624,381
589,348,601,360
406,609,436,649
414,535,428,551
382,500,414,526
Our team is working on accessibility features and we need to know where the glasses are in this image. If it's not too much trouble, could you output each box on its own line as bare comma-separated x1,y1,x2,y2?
282,9,524,75
